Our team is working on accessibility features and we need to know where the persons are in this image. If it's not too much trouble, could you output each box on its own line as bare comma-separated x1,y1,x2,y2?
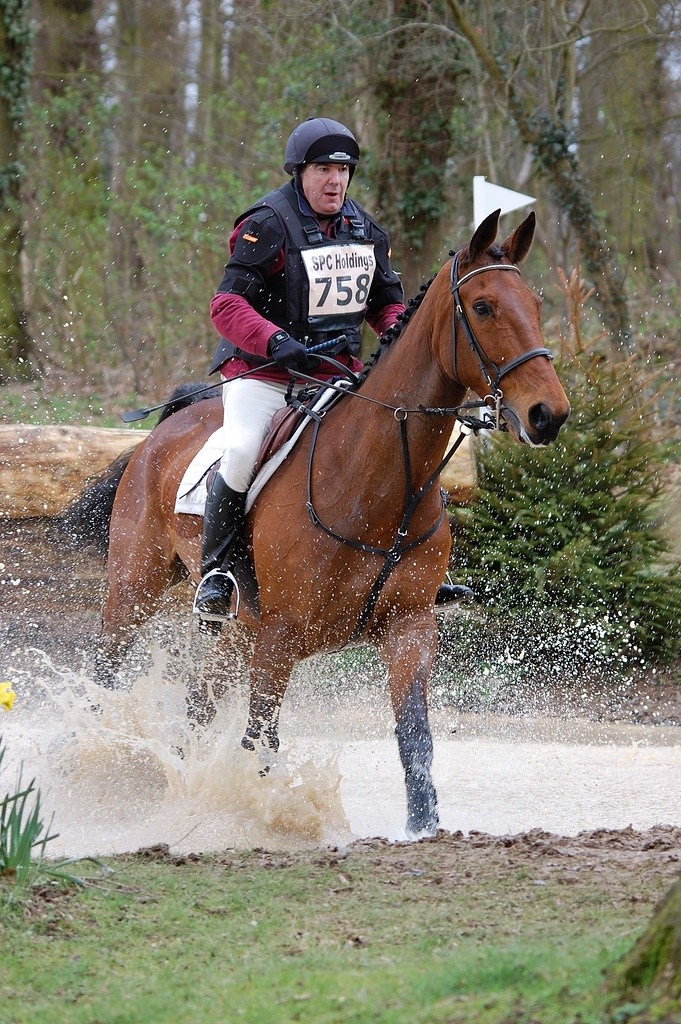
194,116,476,638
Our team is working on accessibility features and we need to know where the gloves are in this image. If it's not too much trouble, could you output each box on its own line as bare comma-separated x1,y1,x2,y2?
268,330,309,372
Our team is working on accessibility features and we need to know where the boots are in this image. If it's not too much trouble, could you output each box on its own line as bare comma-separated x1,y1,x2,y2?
195,471,252,636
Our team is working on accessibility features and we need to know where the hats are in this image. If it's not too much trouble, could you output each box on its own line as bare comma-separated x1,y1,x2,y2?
310,151,359,164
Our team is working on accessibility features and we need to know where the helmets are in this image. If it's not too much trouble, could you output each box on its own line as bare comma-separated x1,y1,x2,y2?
284,117,360,183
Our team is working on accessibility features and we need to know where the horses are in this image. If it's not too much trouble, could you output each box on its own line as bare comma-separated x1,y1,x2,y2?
53,209,571,831
